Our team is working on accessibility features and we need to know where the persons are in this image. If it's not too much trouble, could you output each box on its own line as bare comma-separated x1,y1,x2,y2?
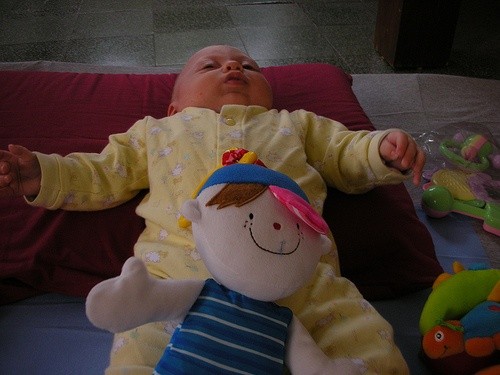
0,44,425,375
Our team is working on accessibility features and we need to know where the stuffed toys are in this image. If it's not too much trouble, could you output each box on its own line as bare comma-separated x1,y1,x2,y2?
84,147,362,375
420,261,500,375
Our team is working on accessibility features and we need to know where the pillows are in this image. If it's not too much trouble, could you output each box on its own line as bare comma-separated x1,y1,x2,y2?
0,62,446,308
